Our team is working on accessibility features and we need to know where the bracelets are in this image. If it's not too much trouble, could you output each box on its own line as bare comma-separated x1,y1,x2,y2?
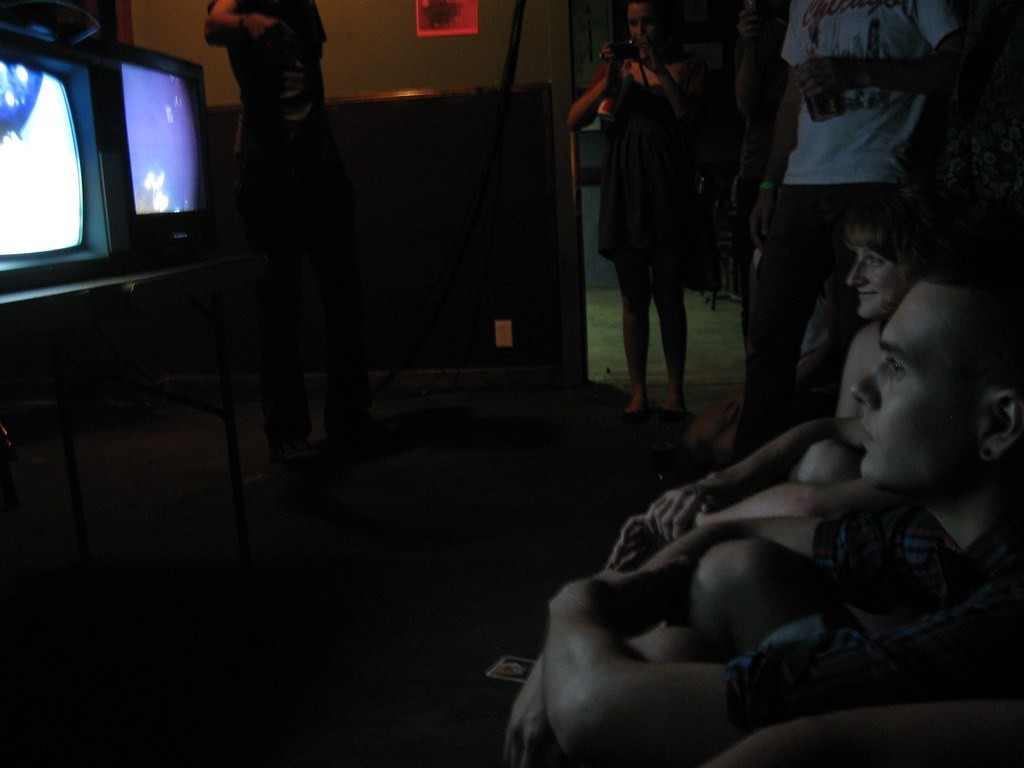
661,542,687,571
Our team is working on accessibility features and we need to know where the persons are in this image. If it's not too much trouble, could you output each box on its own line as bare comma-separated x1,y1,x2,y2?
502,190,1023,768
565,1,710,420
202,1,372,494
723,0,792,302
739,0,983,453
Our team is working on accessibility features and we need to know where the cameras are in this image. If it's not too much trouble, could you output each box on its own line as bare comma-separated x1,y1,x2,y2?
611,40,640,62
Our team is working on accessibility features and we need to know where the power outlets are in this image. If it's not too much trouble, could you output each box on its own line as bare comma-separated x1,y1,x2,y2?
494,319,514,349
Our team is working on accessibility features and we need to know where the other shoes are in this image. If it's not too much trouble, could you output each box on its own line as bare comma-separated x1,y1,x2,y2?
327,421,382,453
658,402,688,422
269,435,319,463
621,403,650,424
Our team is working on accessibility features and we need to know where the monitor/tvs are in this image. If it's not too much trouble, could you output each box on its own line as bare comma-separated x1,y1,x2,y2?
0,27,215,295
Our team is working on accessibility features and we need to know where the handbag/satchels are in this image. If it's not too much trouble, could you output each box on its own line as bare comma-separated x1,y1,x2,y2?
680,168,720,294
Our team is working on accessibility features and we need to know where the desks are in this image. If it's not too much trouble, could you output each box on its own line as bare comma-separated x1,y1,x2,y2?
0,256,265,607
